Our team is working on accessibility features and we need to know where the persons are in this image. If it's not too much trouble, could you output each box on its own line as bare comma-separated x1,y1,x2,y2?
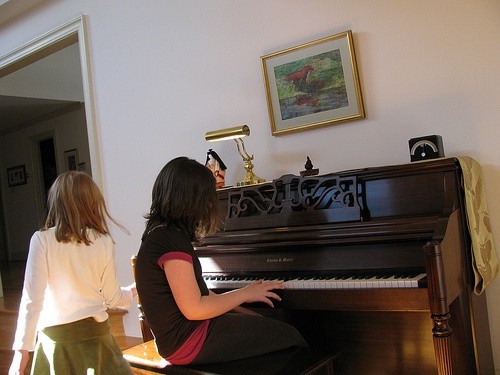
135,156,313,364
203,149,226,189
5,170,140,374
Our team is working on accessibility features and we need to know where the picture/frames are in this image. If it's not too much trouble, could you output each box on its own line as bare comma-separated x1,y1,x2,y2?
63,147,80,171
260,30,368,137
6,164,28,186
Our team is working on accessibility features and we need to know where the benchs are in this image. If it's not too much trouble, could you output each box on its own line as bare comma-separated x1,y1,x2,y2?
121,337,341,375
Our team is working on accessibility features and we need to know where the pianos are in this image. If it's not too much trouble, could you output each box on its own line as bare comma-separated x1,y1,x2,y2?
130,156,496,375
